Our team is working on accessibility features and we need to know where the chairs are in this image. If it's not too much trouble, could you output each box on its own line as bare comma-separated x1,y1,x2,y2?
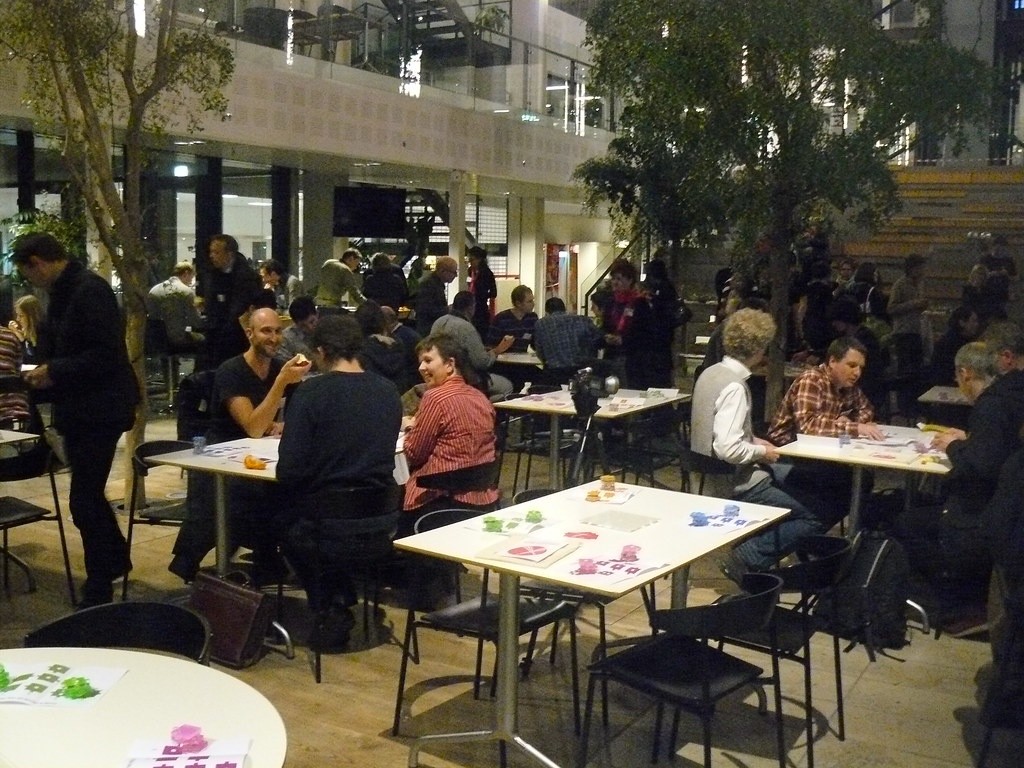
213,5,356,68
1,313,1024,768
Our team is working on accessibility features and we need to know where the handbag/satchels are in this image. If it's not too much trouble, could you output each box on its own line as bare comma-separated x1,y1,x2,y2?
859,286,876,318
167,555,200,583
187,570,277,667
660,299,693,330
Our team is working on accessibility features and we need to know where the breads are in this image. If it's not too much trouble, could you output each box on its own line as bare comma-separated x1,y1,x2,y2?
244,455,266,469
295,353,309,366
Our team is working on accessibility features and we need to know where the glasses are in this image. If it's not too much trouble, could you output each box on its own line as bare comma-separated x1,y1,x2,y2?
442,268,457,274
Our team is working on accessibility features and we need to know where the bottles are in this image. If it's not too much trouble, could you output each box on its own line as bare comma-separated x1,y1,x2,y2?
600,474,615,492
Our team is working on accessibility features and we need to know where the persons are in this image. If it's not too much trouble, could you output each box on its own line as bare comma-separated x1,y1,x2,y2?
150,233,305,374
699,238,771,375
467,246,496,335
766,336,876,563
431,293,513,403
277,315,403,649
313,248,457,393
390,333,499,608
895,346,1018,635
982,319,1024,376
589,258,680,442
0,296,45,426
788,223,1011,421
206,308,312,580
691,308,827,585
9,232,141,609
483,286,601,435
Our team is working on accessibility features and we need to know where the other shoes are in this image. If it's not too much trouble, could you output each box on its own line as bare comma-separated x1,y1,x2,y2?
930,592,1007,638
714,550,756,585
268,604,356,654
510,439,538,451
107,542,133,580
495,437,519,451
76,581,114,614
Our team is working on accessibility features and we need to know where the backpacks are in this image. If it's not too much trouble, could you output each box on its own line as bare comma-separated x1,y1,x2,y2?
812,521,909,650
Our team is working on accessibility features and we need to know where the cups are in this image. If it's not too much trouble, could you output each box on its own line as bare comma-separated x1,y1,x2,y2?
244,454,266,469
192,436,206,454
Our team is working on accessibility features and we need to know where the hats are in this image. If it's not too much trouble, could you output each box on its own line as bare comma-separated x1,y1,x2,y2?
904,253,929,268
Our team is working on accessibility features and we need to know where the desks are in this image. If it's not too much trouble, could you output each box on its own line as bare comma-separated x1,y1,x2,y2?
497,352,542,368
142,432,410,657
393,478,792,768
0,648,287,768
918,387,969,428
493,387,691,490
0,429,41,592
294,13,385,72
772,426,963,633
